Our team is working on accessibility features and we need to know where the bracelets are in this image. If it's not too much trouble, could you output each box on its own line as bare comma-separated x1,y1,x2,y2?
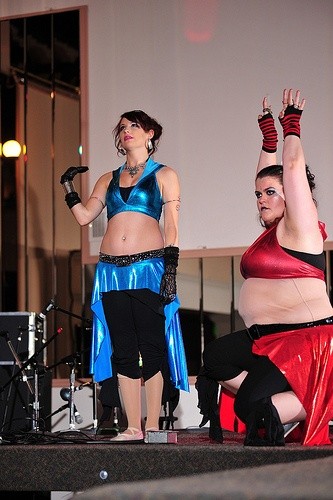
62,181,75,194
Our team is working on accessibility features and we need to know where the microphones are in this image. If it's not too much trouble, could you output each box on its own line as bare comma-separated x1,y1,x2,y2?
69,399,82,424
37,299,55,322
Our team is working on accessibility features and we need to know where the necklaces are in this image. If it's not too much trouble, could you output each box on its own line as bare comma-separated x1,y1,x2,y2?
123,156,150,178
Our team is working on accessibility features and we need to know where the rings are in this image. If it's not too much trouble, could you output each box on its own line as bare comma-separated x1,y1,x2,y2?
294,103,301,107
263,105,273,113
282,101,287,104
288,99,294,105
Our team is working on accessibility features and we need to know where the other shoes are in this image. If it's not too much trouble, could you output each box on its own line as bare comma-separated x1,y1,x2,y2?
109,427,143,441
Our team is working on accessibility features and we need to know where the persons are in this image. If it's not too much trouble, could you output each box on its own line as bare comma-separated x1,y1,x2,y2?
195,89,333,447
60,110,190,441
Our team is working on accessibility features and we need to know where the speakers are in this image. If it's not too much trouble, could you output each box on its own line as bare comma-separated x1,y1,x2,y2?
0,364,52,441
